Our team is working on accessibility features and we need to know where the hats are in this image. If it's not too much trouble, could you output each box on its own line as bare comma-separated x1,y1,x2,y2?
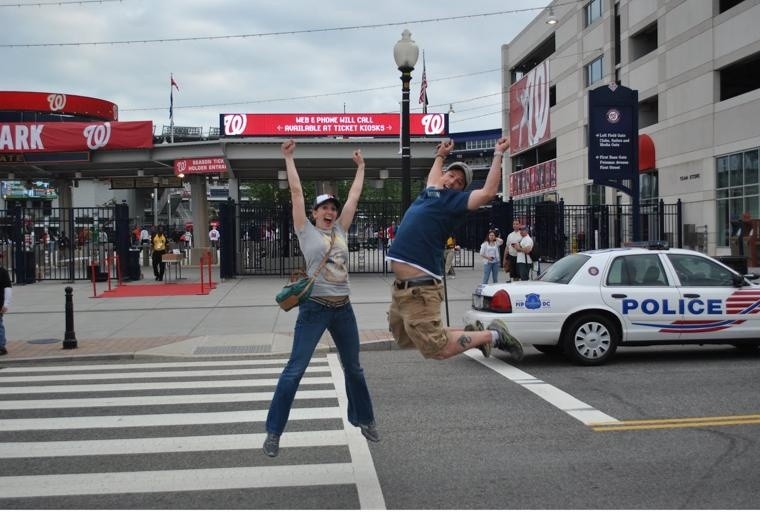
519,225,528,230
312,194,341,209
446,162,473,191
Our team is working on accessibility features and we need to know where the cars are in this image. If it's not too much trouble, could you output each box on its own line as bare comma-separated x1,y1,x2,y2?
463,246,760,365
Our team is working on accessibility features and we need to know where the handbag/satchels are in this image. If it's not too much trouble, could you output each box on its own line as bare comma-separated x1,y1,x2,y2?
275,226,335,313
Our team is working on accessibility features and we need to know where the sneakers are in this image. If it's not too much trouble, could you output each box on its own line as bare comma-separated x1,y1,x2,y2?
469,319,491,358
486,319,524,362
348,418,381,442
263,433,280,457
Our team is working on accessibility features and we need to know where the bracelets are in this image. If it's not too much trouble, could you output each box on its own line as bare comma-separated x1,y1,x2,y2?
436,153,444,158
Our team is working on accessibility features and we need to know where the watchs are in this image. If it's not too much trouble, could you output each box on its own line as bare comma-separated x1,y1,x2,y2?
493,151,503,158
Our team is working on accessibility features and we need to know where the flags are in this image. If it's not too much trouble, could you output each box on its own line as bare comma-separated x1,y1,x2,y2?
172,79,179,92
419,58,428,105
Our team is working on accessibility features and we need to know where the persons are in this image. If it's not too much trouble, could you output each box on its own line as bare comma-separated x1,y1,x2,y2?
209,226,220,250
480,230,503,285
385,137,524,363
445,233,456,279
263,141,382,457
512,225,533,281
0,253,12,355
387,221,398,246
366,224,375,250
151,224,170,281
488,223,501,239
24,224,149,257
504,219,521,283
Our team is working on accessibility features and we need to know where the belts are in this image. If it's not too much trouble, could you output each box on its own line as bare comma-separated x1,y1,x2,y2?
393,279,441,289
308,295,350,308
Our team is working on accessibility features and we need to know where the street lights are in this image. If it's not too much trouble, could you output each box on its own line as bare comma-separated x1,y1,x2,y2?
394,29,419,215
